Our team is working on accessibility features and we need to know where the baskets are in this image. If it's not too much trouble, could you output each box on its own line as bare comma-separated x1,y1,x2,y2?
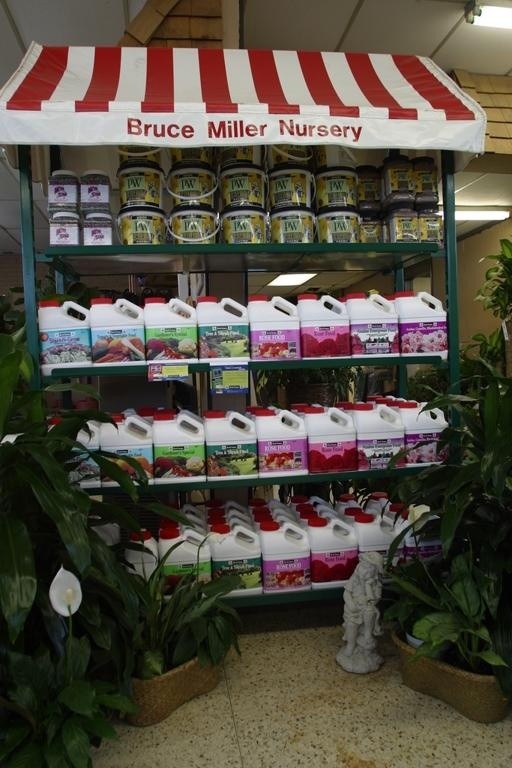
122,651,220,728
390,620,511,723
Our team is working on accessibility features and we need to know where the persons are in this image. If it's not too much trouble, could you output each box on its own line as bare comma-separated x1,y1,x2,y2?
342,552,385,658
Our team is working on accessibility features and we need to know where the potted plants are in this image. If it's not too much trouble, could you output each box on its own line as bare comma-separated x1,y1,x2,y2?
92,531,250,731
367,502,511,728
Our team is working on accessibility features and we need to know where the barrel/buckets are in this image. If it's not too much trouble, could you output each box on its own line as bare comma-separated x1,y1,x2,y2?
114,159,363,244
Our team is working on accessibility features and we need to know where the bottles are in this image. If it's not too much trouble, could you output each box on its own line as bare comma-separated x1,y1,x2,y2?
46,395,450,485
89,489,444,599
35,290,449,366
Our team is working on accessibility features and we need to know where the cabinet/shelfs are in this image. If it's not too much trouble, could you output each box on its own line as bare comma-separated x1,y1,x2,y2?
1,39,488,612
